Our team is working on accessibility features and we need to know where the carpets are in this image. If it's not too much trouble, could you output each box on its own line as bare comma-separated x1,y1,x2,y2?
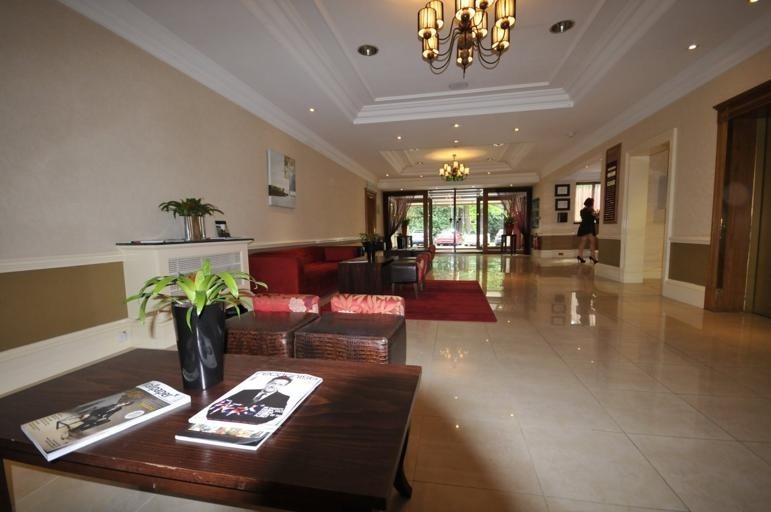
315,279,497,322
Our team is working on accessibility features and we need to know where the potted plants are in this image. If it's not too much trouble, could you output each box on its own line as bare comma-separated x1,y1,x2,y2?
401,218,411,235
158,197,224,241
121,255,267,392
504,214,518,234
360,232,380,263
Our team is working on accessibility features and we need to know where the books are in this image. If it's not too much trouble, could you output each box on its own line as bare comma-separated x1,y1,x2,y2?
18,379,192,461
186,369,324,427
175,423,271,452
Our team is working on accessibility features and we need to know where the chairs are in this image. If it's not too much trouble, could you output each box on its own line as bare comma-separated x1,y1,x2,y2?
292,291,406,364
223,291,322,358
388,244,436,299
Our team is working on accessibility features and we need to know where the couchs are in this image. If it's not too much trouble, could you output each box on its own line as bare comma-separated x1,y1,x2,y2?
248,245,359,303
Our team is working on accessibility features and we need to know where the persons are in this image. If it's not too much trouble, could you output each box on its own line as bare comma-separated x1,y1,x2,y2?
576,266,594,326
209,376,291,422
575,198,598,264
69,400,131,434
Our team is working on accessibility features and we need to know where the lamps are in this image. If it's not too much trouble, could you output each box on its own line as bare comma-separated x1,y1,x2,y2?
439,154,470,181
418,0,517,78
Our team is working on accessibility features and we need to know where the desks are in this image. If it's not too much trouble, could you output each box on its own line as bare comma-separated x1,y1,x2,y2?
336,253,398,295
501,234,516,253
397,235,413,249
0,347,424,512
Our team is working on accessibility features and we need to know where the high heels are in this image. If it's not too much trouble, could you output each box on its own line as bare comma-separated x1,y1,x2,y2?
577,256,585,263
589,256,597,264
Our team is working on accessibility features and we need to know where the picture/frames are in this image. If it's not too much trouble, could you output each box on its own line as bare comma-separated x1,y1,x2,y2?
555,198,570,211
555,184,570,196
214,219,231,238
